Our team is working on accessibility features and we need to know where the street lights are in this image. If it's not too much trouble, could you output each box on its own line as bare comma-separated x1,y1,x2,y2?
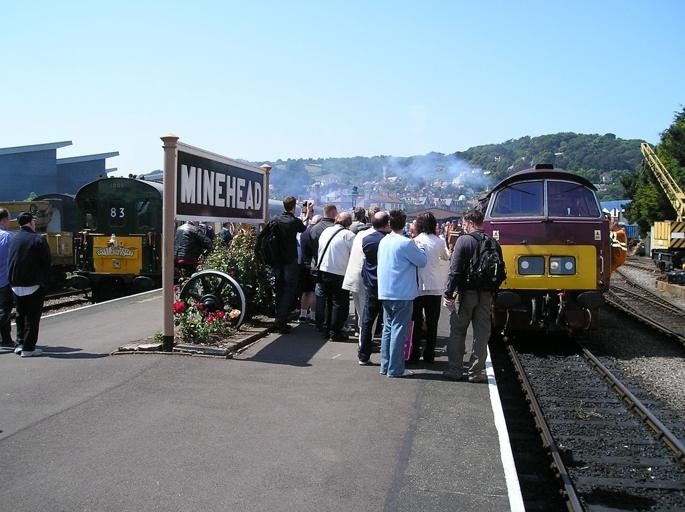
351,186,359,220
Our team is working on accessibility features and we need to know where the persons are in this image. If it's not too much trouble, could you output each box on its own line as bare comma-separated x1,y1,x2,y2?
0,207,15,353
404,220,416,238
358,211,391,365
175,218,234,291
442,210,504,381
266,196,380,342
436,215,469,252
377,209,427,377
8,212,51,357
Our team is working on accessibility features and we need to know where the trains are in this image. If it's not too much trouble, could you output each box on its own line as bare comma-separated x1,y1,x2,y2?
28,193,76,281
62,177,325,305
471,162,616,341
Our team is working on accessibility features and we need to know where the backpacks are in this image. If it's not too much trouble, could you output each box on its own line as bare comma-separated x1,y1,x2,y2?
464,233,506,288
255,220,281,264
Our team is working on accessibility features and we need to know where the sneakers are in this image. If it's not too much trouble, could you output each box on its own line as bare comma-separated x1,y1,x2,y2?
14,348,43,356
387,369,413,377
330,331,346,341
297,315,316,325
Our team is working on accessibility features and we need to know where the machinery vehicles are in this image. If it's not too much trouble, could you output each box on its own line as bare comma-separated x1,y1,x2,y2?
640,142,685,285
